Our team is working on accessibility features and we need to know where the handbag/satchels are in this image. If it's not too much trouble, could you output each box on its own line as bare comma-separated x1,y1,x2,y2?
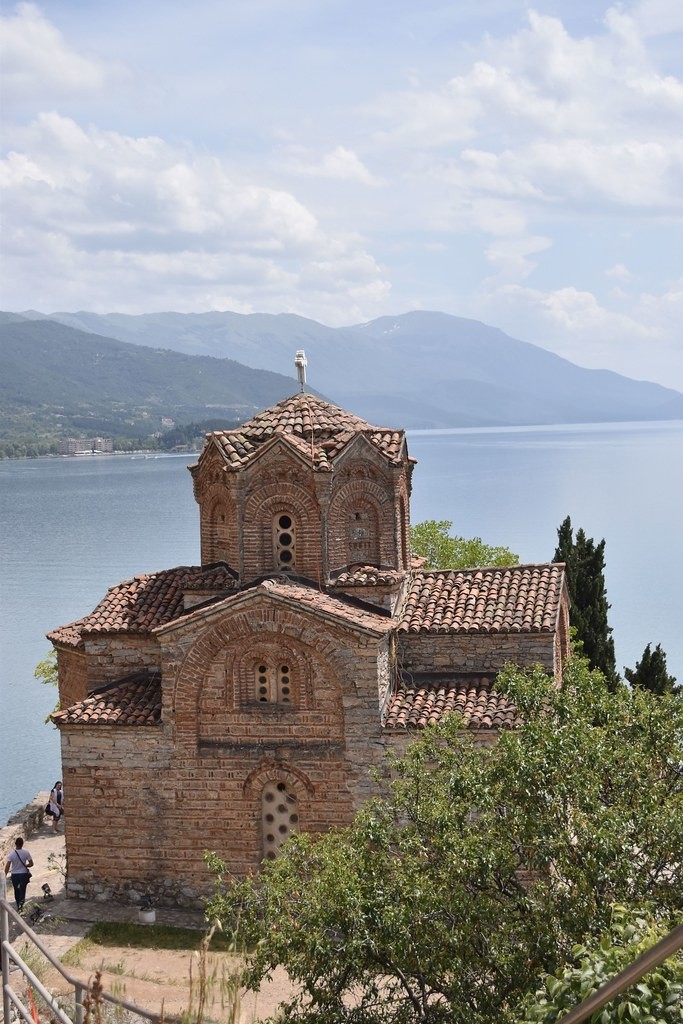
27,870,32,878
46,804,54,815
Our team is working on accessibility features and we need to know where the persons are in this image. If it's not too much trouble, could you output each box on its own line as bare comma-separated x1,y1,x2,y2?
4,837,34,913
49,780,63,834
